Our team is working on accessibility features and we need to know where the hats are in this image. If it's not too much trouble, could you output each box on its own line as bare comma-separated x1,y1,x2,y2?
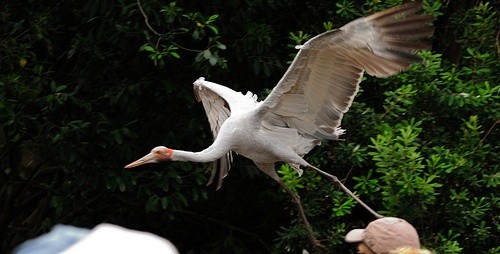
345,217,419,254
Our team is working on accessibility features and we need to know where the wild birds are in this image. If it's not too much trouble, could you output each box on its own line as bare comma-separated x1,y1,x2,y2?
123,0,436,245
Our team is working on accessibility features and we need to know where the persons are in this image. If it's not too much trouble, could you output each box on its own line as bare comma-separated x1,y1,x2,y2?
345,218,419,254
